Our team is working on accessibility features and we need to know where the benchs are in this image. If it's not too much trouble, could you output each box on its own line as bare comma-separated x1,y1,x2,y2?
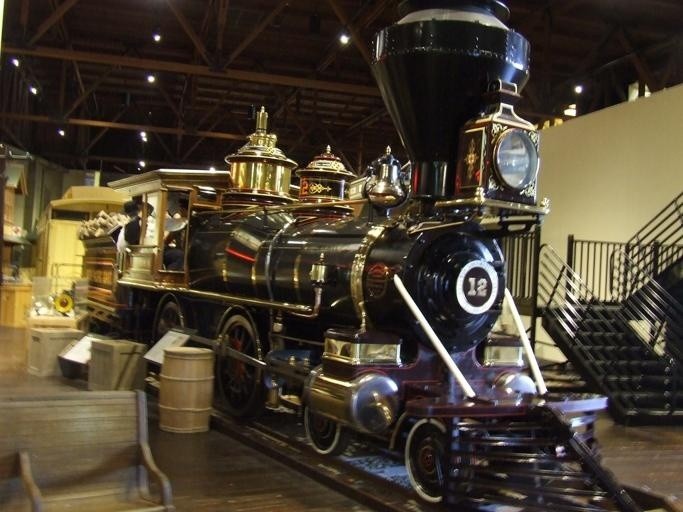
0,387,177,511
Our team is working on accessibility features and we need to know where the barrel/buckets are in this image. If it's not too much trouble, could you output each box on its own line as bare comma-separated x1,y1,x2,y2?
156,346,216,433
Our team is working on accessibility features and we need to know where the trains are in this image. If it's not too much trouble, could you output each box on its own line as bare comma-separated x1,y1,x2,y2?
54,1,639,512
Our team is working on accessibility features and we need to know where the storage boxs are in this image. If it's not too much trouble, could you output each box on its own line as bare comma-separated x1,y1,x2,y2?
30,316,74,327
87,339,147,391
26,327,85,377
